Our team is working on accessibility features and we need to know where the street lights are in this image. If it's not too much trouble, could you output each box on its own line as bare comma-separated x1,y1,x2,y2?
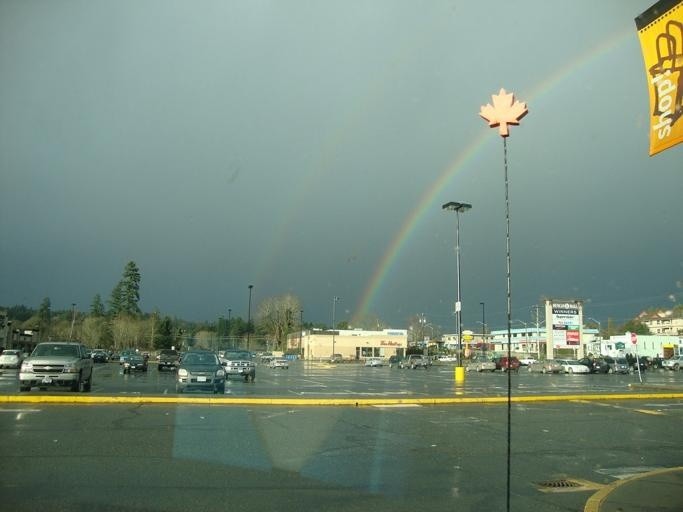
299,310,305,353
479,302,486,355
588,319,601,354
247,285,253,349
227,309,232,336
442,201,471,384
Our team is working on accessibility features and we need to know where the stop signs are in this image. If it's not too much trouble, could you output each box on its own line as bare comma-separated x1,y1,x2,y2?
631,333,637,344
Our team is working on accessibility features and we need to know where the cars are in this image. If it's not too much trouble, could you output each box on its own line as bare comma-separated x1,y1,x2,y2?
120,350,149,373
519,357,536,365
0,349,23,368
90,350,109,363
365,357,383,367
388,355,401,367
261,352,289,370
528,359,590,375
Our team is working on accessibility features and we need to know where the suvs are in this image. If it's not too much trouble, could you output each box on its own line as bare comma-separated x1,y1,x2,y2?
662,355,683,371
466,355,520,373
18,341,93,392
158,349,179,370
220,350,255,380
177,350,227,395
628,358,648,372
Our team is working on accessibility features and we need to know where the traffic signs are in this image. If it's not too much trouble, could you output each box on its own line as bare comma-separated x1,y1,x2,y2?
514,318,545,357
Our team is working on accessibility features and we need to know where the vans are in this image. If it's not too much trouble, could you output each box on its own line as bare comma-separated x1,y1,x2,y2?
578,356,629,374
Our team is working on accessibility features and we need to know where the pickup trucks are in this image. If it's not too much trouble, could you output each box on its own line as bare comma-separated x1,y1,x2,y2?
398,354,432,369
